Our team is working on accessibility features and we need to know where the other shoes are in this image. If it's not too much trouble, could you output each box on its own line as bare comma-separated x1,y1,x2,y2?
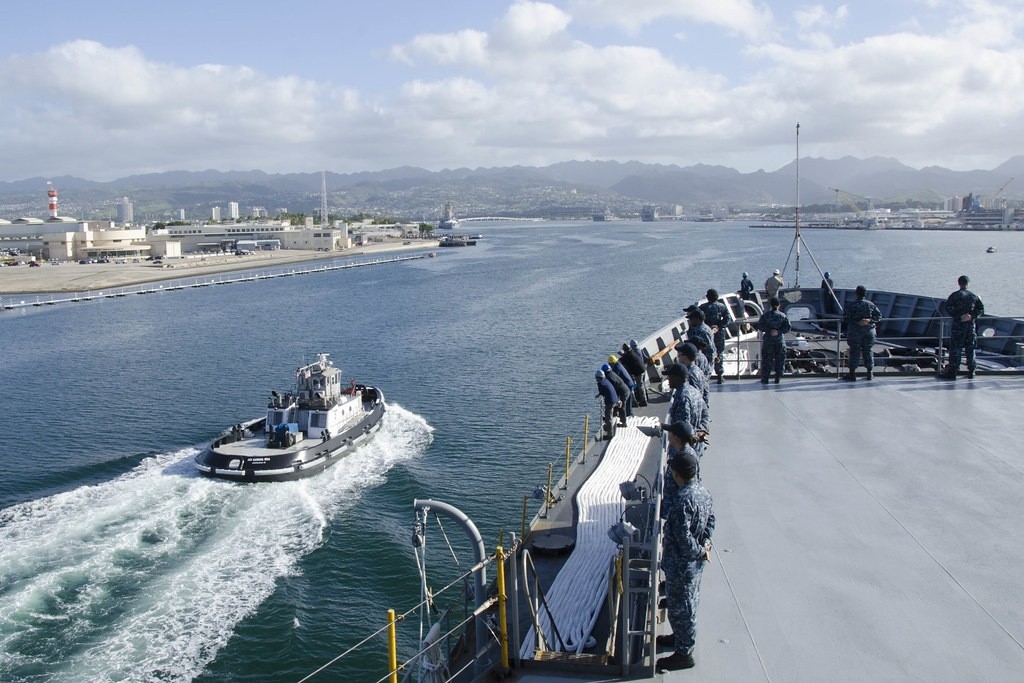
718,377,725,384
657,634,676,647
939,372,955,380
657,652,694,668
843,375,856,381
867,374,874,380
774,375,780,383
968,371,975,379
761,377,767,383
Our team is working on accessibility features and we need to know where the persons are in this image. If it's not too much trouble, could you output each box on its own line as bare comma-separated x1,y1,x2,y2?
606,354,635,417
700,288,731,384
661,363,710,459
400,234,461,239
684,336,712,382
683,305,721,371
942,275,984,380
630,339,648,401
656,451,716,671
601,364,630,427
595,369,618,440
821,271,834,312
674,342,709,408
758,297,792,384
741,272,754,309
764,268,784,310
658,418,701,607
842,285,882,382
685,308,714,364
618,342,650,406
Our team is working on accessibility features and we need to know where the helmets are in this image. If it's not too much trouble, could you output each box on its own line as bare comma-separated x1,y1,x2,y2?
825,272,830,277
743,272,748,277
773,269,780,275
595,339,638,377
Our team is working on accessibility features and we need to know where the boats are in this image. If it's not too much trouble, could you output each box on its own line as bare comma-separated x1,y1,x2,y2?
193,352,387,485
438,233,477,247
986,246,998,254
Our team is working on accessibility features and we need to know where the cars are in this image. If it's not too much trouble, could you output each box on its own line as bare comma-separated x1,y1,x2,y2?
0,246,22,266
78,255,166,265
30,262,41,267
52,261,59,265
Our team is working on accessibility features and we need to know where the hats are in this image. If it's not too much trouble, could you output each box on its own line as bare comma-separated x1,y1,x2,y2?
857,286,866,295
770,298,779,306
686,309,705,320
683,305,697,312
675,343,697,359
668,453,696,474
706,289,717,299
662,363,689,378
662,420,691,440
959,275,969,284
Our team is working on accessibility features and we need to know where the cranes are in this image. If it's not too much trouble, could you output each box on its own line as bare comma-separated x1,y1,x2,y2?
829,185,877,224
991,176,1015,208
